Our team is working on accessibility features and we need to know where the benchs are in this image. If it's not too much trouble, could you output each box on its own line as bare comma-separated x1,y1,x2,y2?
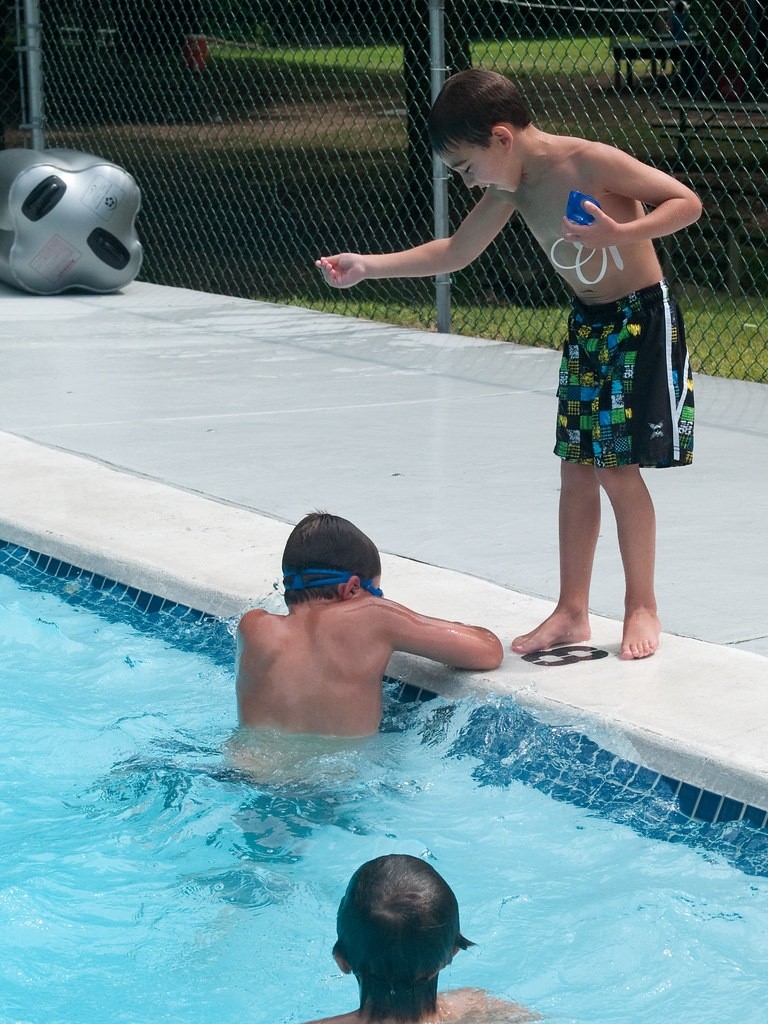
652,119,768,132
658,129,768,145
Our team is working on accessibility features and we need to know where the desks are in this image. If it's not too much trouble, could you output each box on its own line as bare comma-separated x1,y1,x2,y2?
657,102,768,159
612,40,697,96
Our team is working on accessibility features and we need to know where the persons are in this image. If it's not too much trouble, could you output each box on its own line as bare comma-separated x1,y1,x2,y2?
315,66,706,662
232,513,504,734
302,853,542,1024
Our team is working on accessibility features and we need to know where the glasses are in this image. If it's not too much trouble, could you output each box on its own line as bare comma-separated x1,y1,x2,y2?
364,580,384,598
